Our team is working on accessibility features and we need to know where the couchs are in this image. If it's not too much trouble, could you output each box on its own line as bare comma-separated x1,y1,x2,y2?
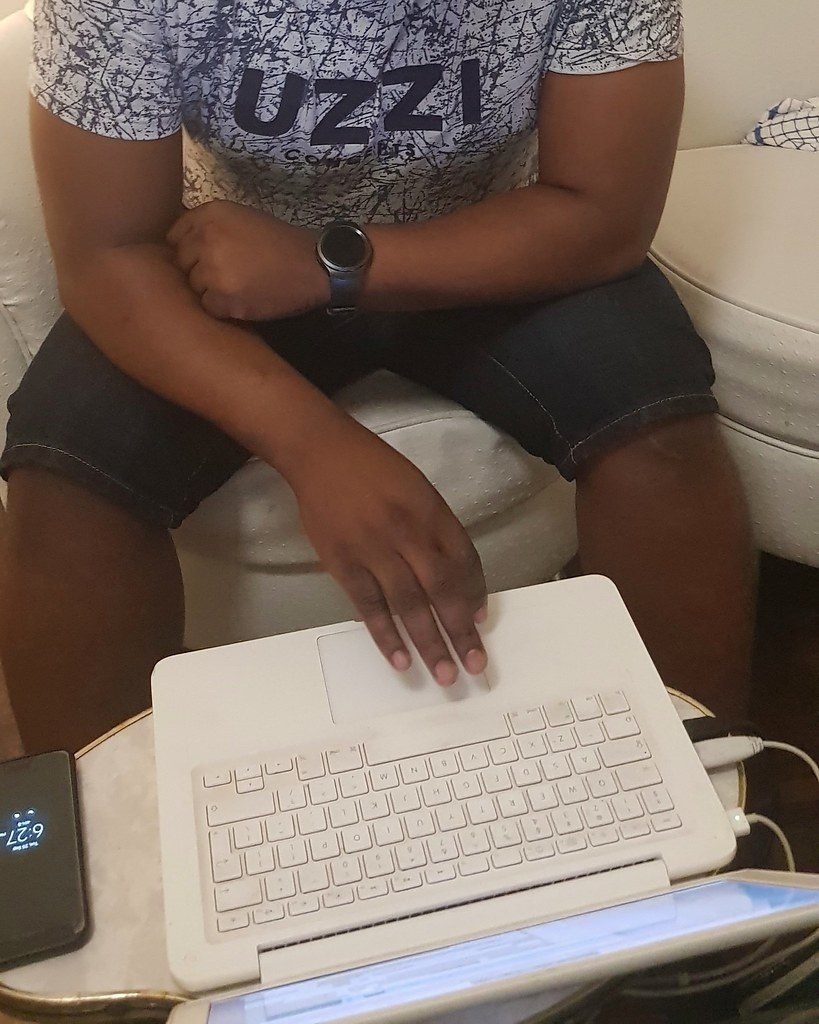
0,2,819,658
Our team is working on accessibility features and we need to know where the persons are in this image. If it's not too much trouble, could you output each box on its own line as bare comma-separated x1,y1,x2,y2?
0,0,754,758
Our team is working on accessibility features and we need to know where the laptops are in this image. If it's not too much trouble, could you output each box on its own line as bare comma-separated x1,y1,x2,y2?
149,573,819,1024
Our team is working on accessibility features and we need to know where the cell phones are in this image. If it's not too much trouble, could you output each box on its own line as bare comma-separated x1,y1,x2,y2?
1,746,91,973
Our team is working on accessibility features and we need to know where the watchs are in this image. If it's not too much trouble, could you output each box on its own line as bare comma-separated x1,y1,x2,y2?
314,220,374,316
